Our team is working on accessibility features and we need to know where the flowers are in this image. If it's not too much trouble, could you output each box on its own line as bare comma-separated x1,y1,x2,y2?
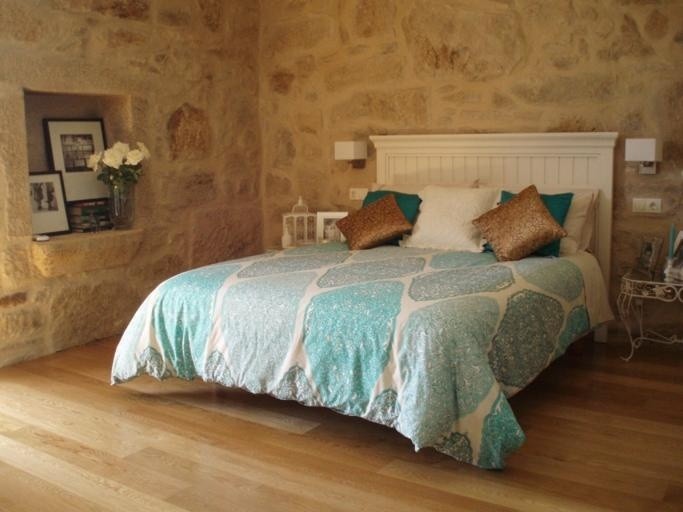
86,141,151,198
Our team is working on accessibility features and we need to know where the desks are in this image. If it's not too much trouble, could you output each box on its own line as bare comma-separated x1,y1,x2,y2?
616,271,683,361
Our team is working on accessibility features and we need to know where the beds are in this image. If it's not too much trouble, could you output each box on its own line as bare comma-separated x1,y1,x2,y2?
158,131,619,451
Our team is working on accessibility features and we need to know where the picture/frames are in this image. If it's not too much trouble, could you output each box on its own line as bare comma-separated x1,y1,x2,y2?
28,118,111,236
317,211,348,243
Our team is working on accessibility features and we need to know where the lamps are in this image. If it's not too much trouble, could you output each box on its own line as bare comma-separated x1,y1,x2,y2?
335,141,367,168
625,137,663,174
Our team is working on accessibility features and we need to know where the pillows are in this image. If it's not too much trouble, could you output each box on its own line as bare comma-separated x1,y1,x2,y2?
334,178,600,261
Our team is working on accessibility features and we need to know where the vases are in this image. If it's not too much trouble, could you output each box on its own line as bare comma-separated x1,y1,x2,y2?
109,184,135,228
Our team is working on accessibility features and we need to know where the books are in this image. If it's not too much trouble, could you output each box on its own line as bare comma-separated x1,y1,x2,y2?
70,216,111,224
69,206,111,216
71,223,112,229
71,226,111,233
662,263,682,281
70,199,108,206
